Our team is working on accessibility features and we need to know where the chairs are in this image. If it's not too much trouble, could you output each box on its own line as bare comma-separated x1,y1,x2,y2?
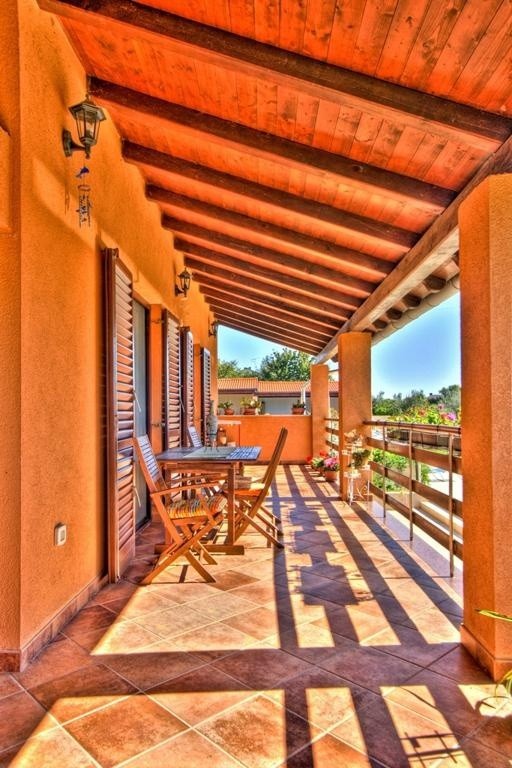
132,425,288,585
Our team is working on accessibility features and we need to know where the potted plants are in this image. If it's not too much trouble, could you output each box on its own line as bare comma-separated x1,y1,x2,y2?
291,399,306,415
218,400,234,415
239,397,264,415
312,457,325,475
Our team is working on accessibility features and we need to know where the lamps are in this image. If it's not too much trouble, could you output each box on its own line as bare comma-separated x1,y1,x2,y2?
175,267,193,298
209,321,219,338
62,93,107,159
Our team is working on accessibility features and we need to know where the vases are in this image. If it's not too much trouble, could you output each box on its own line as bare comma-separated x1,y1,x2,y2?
324,471,336,482
311,465,314,469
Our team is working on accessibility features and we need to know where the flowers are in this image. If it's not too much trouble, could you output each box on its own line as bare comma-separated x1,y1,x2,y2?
304,451,329,464
323,457,341,472
